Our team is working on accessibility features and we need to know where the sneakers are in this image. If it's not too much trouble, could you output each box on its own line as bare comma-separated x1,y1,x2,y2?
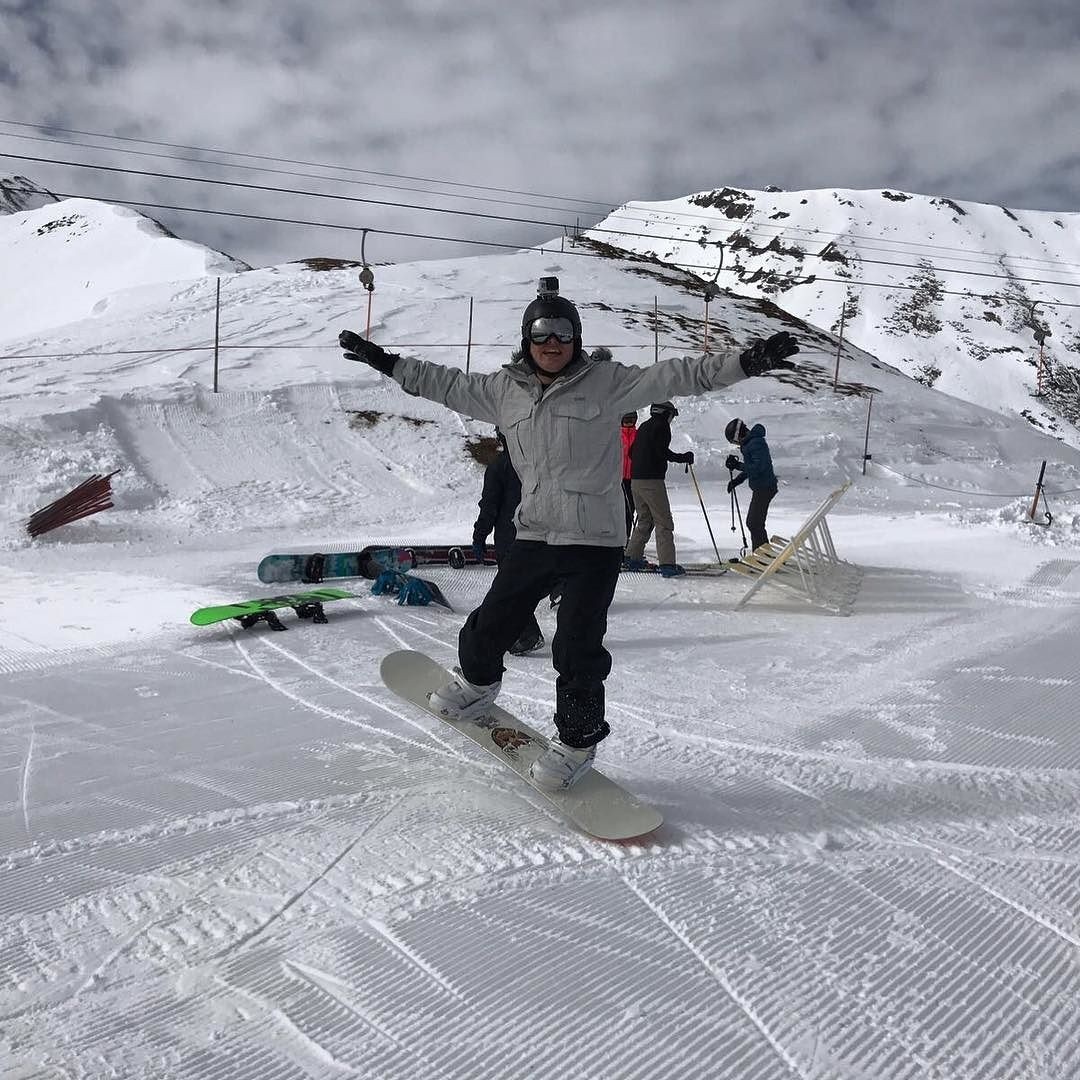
625,558,658,572
528,729,599,789
429,668,501,719
661,563,687,577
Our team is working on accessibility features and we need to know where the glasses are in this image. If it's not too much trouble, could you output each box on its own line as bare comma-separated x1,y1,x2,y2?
528,316,576,344
623,417,636,423
731,440,739,443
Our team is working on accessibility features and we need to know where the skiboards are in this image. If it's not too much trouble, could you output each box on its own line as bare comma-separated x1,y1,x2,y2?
617,563,728,577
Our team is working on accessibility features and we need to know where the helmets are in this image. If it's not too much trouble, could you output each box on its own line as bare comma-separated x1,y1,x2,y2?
520,297,582,339
650,400,678,416
622,411,637,421
725,417,743,444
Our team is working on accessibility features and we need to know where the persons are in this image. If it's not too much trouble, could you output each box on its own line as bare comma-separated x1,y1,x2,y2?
620,400,694,578
471,426,546,657
725,418,777,571
339,296,799,790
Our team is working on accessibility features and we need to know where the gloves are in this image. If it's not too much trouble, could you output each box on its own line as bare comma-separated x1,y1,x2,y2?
740,331,800,377
339,331,400,377
725,456,738,470
472,540,488,564
681,451,695,465
727,480,733,492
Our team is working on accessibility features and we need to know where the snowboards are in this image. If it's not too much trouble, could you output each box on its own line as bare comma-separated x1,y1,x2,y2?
361,544,497,572
190,588,362,627
256,550,414,585
370,567,454,614
380,649,665,842
